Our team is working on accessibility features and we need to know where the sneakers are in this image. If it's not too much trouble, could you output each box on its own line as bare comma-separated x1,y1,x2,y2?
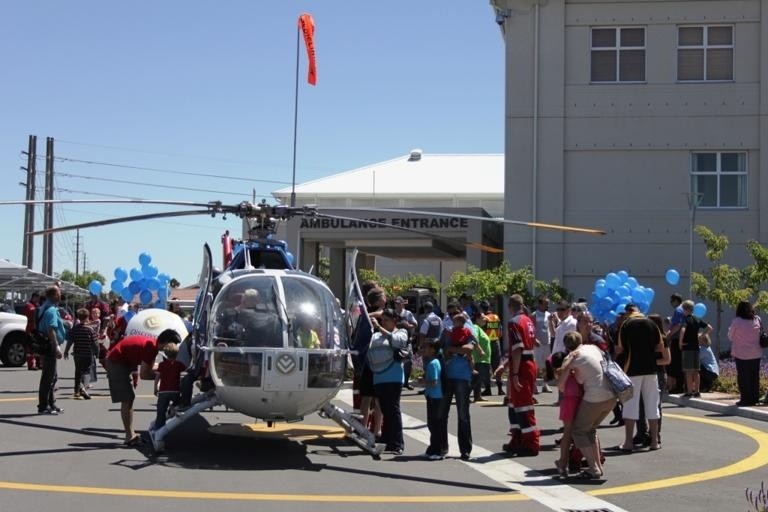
681,393,692,399
51,403,64,413
429,454,446,460
618,443,633,452
460,451,470,459
650,444,663,450
74,391,80,400
694,392,700,399
81,388,91,399
37,404,58,415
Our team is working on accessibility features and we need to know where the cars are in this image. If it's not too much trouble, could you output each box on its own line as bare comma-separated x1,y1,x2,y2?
0,310,29,368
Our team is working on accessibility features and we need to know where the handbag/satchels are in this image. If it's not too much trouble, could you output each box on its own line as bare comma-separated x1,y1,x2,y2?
392,345,414,364
759,332,768,348
599,357,636,406
26,328,51,356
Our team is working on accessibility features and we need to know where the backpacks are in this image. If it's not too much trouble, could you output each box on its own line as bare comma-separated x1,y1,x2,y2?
367,331,393,372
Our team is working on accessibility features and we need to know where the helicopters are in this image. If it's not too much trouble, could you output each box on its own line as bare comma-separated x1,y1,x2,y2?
0,197,605,464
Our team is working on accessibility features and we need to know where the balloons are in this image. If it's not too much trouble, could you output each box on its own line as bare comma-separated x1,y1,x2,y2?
88,251,171,309
588,270,655,327
666,269,680,286
233,231,294,265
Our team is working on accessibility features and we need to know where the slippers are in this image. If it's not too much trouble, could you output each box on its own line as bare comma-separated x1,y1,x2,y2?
574,470,602,482
124,435,139,445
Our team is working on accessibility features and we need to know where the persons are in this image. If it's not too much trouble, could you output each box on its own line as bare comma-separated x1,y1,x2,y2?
503,294,720,478
237,287,282,346
415,287,506,461
23,286,193,447
296,315,320,350
349,280,418,454
728,300,761,407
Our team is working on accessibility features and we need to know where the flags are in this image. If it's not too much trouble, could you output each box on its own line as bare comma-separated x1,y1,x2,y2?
299,11,317,86
